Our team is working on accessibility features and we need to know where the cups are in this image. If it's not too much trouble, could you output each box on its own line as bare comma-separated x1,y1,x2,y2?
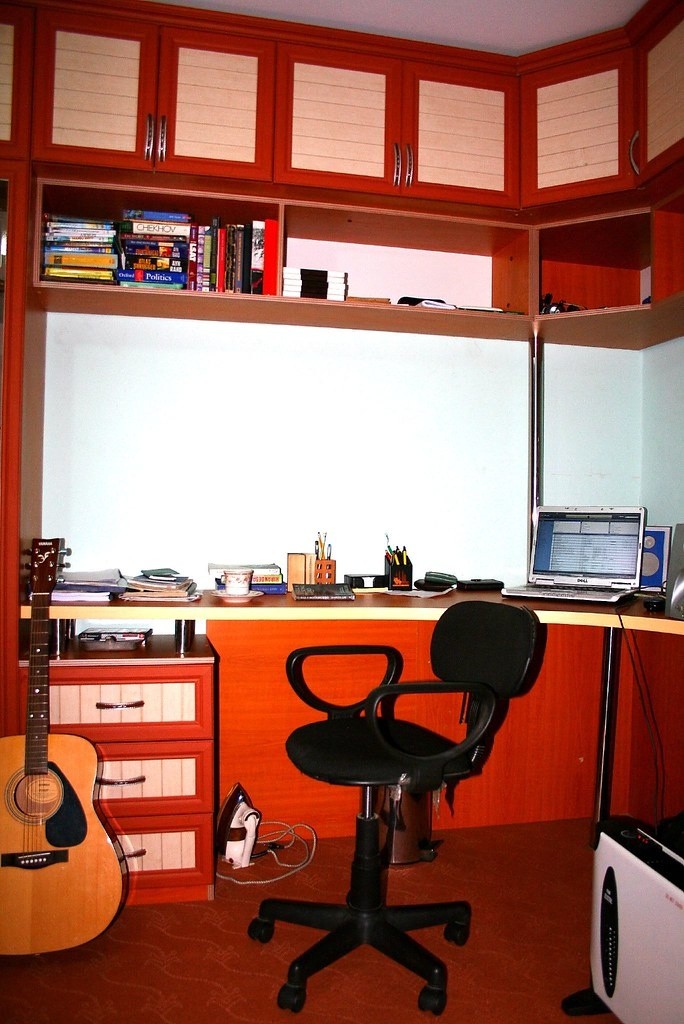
224,570,254,595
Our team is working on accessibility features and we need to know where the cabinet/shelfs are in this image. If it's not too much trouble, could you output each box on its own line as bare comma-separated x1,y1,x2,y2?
0,0,684,904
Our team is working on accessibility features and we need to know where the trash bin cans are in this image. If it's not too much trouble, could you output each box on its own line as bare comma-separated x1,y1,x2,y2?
370,784,444,866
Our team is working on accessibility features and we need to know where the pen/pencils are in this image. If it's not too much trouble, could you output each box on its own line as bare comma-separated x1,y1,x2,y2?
385,545,407,566
318,532,327,560
314,540,320,561
326,543,331,560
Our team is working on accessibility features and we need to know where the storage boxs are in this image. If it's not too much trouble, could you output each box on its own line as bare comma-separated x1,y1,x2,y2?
314,559,336,585
384,554,413,592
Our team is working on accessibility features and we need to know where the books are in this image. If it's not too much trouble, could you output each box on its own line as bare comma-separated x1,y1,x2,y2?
51,567,203,602
208,563,287,596
78,628,153,641
281,266,348,302
292,583,356,601
346,296,391,303
40,207,279,295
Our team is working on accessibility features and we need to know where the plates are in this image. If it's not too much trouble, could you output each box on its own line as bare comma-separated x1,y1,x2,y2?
211,590,264,602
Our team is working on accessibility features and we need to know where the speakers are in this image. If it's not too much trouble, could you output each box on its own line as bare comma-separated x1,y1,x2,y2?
665,523,684,620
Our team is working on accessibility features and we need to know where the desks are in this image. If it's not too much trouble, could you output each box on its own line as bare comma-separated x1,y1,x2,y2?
18,586,684,849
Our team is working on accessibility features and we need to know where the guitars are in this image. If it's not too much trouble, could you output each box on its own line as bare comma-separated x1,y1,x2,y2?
0,537,134,959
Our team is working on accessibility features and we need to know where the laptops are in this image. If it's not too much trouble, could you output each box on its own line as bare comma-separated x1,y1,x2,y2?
501,506,649,605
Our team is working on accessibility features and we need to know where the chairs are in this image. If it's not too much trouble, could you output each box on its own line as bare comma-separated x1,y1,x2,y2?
245,599,549,1016
